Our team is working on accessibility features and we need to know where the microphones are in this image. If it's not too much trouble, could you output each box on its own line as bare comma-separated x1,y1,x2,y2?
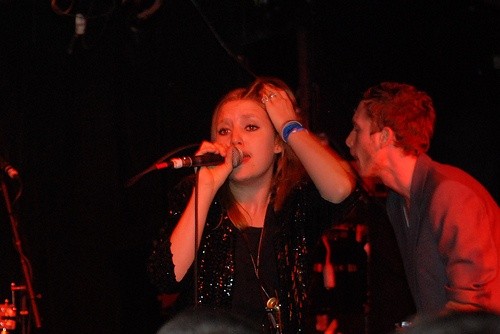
155,147,240,170
0,156,23,186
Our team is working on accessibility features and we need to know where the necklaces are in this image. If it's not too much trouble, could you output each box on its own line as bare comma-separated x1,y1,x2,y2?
225,190,279,313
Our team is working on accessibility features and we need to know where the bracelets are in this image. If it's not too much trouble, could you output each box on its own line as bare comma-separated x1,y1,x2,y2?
281,119,304,145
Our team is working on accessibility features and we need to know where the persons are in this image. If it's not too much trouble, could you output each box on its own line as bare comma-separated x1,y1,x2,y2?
141,75,387,334
345,81,500,334
